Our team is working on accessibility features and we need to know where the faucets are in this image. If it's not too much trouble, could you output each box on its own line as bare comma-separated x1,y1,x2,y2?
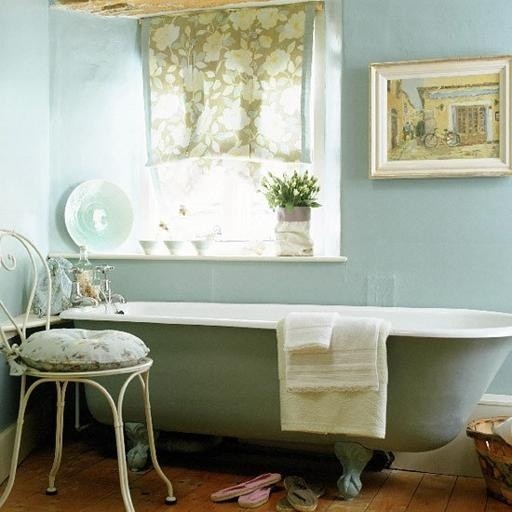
95,264,126,305
67,267,98,307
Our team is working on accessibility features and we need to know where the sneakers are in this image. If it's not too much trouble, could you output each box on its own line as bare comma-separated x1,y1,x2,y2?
210,473,325,511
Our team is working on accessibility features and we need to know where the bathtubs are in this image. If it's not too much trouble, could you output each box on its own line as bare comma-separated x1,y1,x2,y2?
60,299,512,501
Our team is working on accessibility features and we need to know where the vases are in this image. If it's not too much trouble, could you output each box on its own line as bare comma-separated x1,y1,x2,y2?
275,207,314,256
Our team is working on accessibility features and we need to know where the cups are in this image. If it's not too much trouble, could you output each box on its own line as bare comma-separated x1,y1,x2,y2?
139,240,209,257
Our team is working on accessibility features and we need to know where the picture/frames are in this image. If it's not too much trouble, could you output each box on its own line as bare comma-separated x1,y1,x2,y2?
368,54,511,181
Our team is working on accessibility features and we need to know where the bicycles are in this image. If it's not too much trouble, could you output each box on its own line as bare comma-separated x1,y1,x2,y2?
423,127,460,148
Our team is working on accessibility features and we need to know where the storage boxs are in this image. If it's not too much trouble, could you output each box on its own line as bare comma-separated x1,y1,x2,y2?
466,413,511,508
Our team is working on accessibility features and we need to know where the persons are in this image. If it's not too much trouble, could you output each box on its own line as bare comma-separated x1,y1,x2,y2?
403,122,415,140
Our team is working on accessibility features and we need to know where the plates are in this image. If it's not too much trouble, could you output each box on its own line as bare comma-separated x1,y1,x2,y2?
63,179,134,254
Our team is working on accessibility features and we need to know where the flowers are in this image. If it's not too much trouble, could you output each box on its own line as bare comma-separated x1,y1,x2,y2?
254,169,323,210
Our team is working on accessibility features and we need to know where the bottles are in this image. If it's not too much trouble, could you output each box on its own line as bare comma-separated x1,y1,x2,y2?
76,245,93,298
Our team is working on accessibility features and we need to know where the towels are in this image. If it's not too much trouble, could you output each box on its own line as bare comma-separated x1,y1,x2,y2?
276,311,390,440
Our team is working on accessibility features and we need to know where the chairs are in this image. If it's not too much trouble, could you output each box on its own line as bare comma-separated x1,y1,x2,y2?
0,218,177,510
